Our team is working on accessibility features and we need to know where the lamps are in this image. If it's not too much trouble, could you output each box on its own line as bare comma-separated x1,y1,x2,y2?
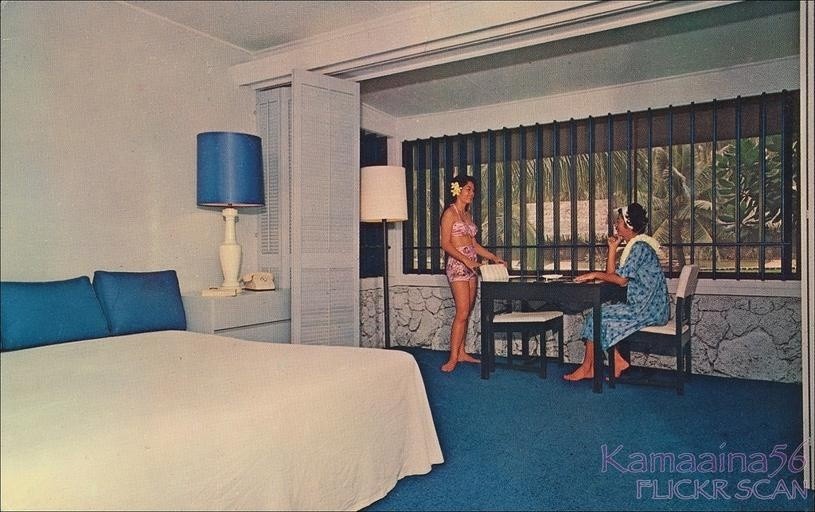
361,165,407,350
196,132,266,296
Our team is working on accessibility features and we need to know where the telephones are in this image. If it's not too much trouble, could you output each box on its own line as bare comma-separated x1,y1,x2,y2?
244,271,275,291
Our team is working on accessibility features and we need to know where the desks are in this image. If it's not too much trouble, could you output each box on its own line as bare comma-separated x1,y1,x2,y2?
479,275,626,394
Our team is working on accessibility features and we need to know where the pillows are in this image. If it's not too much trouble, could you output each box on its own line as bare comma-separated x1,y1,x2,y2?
1,269,188,351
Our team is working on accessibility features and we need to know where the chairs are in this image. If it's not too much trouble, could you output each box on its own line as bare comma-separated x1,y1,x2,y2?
610,263,701,394
477,264,564,379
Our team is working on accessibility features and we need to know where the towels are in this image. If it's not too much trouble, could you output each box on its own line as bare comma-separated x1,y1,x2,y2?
619,233,668,266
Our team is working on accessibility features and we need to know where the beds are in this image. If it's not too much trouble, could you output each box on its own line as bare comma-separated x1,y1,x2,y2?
0,329,446,512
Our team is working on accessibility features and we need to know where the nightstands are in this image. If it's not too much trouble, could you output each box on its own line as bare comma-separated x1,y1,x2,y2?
182,289,289,345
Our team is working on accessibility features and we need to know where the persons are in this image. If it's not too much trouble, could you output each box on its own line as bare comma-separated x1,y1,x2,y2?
562,203,671,381
440,175,508,372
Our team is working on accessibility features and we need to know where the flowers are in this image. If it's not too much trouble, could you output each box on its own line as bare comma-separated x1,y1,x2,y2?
449,182,463,197
